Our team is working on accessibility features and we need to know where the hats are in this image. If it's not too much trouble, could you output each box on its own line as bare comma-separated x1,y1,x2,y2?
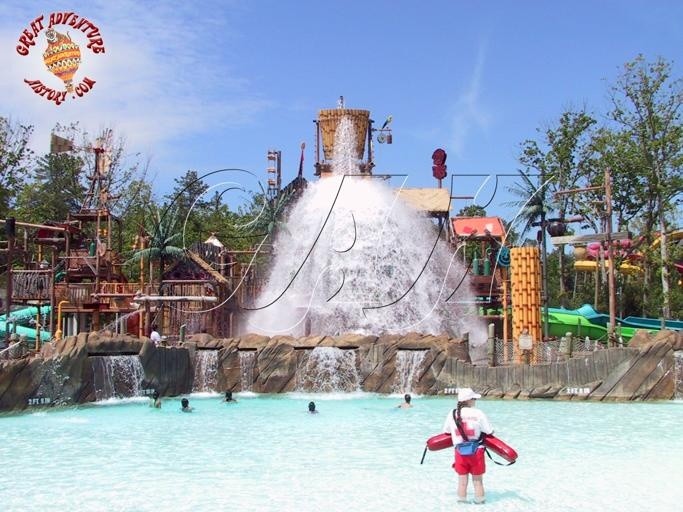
458,388,481,400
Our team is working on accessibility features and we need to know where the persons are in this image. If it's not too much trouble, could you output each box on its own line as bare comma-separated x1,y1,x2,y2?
441,386,494,505
218,389,240,405
147,391,161,408
396,393,417,409
149,324,160,340
304,401,319,415
176,397,192,412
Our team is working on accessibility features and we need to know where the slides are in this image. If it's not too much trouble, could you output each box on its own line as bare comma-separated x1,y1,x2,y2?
473,304,682,347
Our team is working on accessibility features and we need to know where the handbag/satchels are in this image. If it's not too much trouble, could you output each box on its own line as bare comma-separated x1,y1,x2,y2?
456,442,479,455
